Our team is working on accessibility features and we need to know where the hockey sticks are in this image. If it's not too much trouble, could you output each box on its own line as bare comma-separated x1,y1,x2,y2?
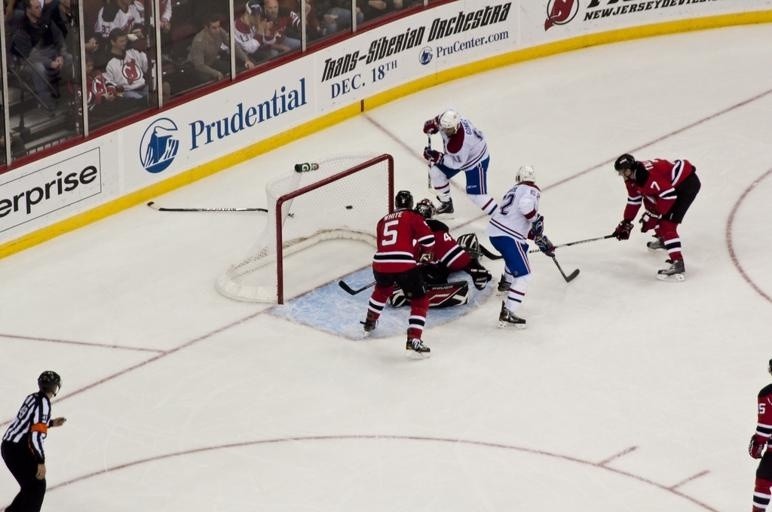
427,133,457,219
147,201,268,214
339,280,376,296
479,235,614,260
553,256,579,282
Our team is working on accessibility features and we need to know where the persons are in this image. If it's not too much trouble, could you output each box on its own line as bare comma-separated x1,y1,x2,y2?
0,370,67,512
750,360,772,512
614,154,701,276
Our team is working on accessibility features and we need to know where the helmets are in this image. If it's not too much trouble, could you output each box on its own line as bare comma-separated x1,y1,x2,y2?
438,109,463,130
614,153,637,174
414,199,434,219
395,190,414,210
38,371,61,388
517,164,536,184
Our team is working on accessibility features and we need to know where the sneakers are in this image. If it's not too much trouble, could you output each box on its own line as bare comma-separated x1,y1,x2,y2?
470,266,491,292
647,234,669,249
657,258,685,276
406,336,431,353
364,319,380,332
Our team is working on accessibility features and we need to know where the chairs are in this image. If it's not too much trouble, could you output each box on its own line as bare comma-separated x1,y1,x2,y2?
177,1,321,90
4,1,179,120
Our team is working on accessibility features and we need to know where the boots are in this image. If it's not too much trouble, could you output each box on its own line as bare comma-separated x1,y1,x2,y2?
498,274,512,291
434,196,454,214
498,301,526,325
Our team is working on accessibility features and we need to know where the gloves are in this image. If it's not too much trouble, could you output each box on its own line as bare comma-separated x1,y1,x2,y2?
423,147,446,167
423,118,439,135
419,252,434,267
534,236,557,259
639,212,660,234
533,216,546,238
613,219,635,241
748,436,766,460
